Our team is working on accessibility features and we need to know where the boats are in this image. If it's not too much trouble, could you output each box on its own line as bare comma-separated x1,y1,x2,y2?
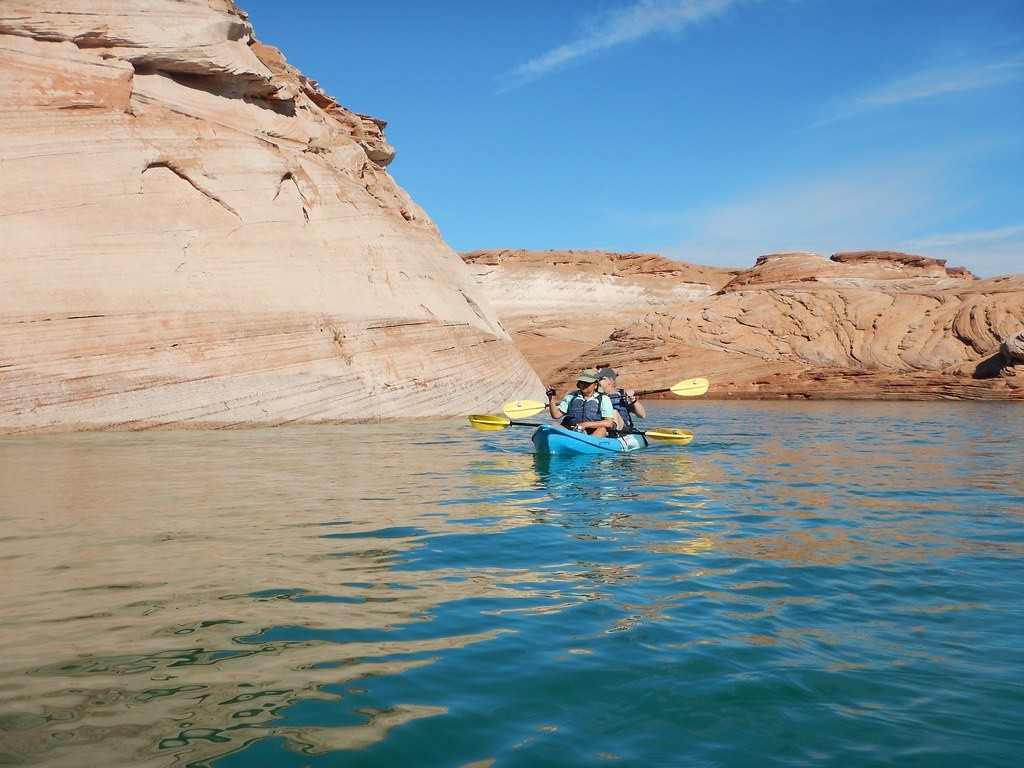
531,452,624,500
531,425,649,455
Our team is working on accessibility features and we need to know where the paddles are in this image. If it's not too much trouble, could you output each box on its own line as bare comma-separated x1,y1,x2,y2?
469,414,693,445
503,378,709,419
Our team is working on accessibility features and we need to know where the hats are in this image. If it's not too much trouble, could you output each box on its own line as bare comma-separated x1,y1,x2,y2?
578,369,599,383
598,368,616,378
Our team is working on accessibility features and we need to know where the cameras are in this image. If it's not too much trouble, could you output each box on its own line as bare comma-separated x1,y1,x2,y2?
546,389,556,397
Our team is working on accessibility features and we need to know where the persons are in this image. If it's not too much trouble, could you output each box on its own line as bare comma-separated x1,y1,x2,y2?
596,367,646,437
546,369,614,439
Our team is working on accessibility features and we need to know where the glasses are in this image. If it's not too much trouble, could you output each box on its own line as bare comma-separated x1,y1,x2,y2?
598,377,606,380
578,382,592,388
